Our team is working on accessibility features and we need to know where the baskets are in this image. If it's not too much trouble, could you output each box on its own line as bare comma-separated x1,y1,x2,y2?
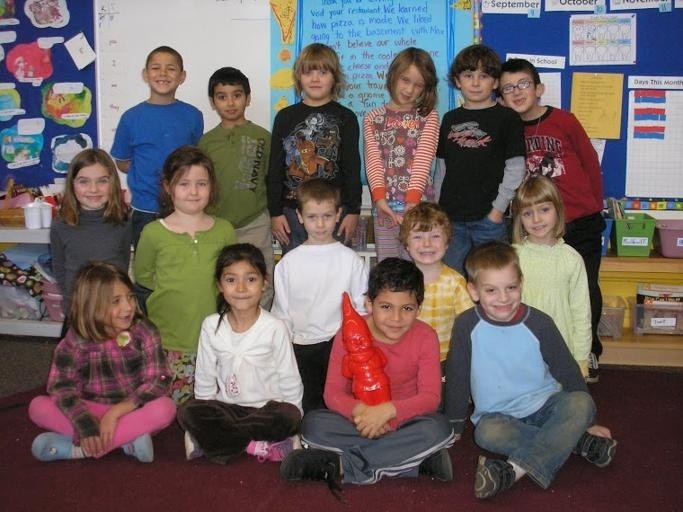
0,177,25,226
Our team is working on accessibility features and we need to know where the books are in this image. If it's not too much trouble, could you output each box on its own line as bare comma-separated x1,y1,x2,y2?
609,196,628,219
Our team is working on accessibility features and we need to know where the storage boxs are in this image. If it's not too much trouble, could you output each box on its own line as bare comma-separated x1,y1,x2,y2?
614,214,656,258
602,217,612,257
599,294,626,340
637,284,683,330
655,219,670,257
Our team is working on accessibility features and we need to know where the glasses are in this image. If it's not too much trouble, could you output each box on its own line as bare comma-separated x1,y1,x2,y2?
501,81,530,94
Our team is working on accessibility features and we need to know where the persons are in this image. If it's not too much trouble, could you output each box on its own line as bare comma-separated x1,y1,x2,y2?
396,200,476,374
276,258,452,484
439,241,617,499
27,263,178,462
510,175,592,386
181,240,301,465
339,291,392,407
108,46,205,318
496,58,606,385
131,143,238,414
194,66,274,315
270,176,368,414
362,47,440,264
51,149,132,338
438,44,526,279
264,42,361,257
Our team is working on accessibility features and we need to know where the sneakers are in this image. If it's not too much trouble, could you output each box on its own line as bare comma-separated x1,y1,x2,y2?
474,456,516,498
32,432,72,462
258,435,302,464
418,450,453,482
586,353,600,382
185,431,204,461
121,435,153,462
573,431,618,470
279,450,345,502
